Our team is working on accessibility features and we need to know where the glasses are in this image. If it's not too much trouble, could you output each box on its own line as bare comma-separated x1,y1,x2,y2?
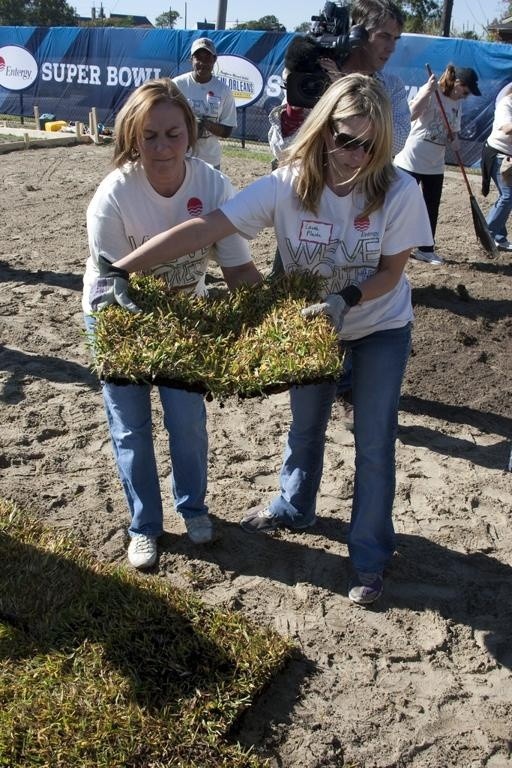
328,120,376,154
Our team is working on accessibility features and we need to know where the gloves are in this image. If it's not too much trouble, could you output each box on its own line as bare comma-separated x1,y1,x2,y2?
298,286,361,334
89,255,145,320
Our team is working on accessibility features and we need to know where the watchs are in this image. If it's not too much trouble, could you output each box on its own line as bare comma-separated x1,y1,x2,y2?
343,283,365,310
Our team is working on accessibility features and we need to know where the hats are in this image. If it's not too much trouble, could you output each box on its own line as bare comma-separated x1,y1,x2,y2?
192,38,216,56
454,67,482,96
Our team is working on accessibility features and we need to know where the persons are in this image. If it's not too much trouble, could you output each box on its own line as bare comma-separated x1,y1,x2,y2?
268,0,410,167
90,78,268,569
393,64,482,266
171,37,237,171
492,79,512,104
486,95,512,250
91,70,435,606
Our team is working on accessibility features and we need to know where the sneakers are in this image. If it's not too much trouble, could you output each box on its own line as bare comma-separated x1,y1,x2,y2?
239,504,315,534
347,570,384,604
128,534,158,570
410,247,444,266
184,513,214,545
494,238,512,251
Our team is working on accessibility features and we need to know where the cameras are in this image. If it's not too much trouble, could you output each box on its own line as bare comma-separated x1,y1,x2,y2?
286,0,369,109
